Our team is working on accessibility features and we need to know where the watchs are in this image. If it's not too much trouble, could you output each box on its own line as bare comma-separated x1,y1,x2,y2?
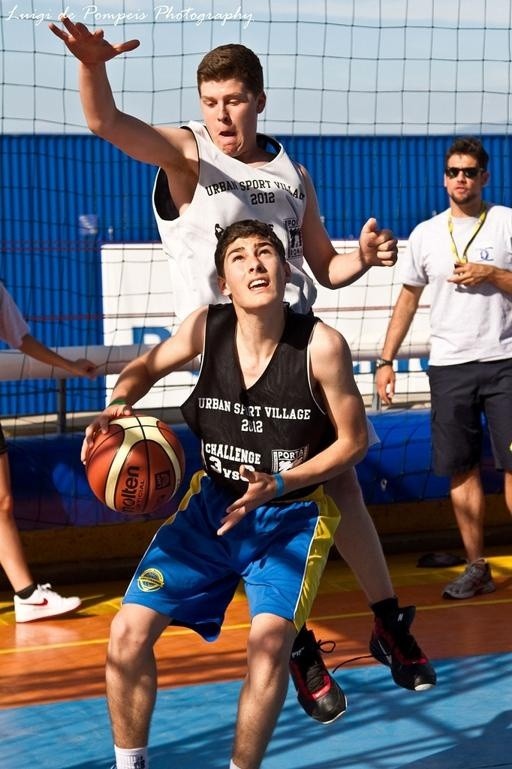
376,357,393,367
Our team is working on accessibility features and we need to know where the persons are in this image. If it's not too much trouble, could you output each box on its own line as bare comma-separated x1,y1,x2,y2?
374,139,512,599
80,219,369,769
49,15,437,724
0,282,97,624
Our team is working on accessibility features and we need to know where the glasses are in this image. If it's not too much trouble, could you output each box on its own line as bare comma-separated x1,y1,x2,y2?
445,167,485,178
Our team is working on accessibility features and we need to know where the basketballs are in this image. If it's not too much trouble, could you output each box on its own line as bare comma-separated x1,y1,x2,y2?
85,413,186,515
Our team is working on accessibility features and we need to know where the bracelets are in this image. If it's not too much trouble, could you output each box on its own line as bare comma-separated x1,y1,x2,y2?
274,474,284,497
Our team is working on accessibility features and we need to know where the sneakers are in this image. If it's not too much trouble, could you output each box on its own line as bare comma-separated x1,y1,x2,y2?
13,583,82,623
288,629,347,724
369,605,437,692
442,561,496,600
